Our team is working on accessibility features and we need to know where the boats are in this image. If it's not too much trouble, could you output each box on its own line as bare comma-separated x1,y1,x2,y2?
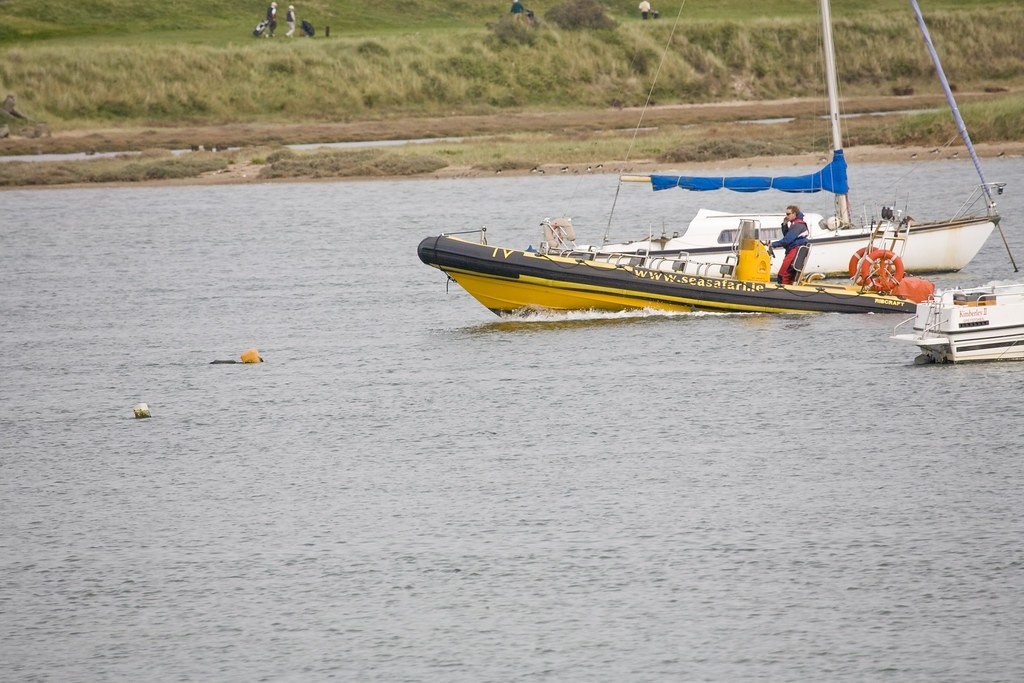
887,279,1024,362
413,226,925,319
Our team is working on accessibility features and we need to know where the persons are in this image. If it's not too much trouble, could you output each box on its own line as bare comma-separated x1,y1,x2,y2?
267,1,296,39
510,0,535,28
765,205,809,285
639,0,651,20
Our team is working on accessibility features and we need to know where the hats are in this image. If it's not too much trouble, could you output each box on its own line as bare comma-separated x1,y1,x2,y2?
271,2,278,5
289,5,294,9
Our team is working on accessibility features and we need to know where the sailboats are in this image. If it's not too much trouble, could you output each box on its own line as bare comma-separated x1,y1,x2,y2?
568,0,1018,280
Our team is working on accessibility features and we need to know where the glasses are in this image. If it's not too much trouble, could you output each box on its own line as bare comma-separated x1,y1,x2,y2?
786,213,794,215
